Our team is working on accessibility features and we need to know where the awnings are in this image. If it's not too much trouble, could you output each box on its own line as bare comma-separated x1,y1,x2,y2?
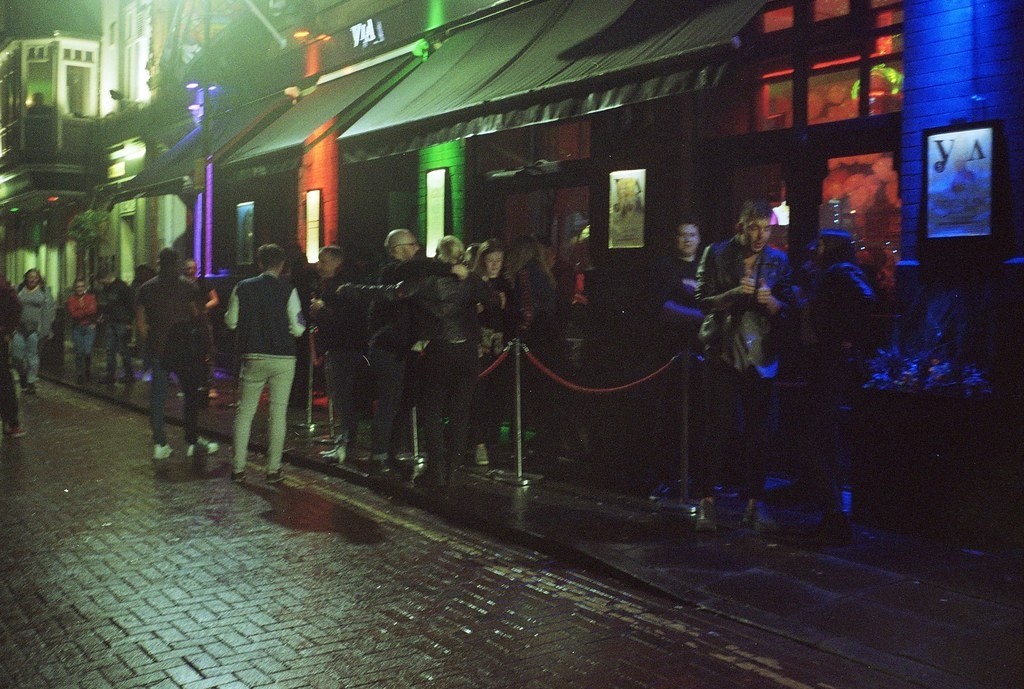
112,0,770,201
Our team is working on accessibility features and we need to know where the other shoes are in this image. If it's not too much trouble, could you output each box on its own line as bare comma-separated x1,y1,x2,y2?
2,422,27,438
231,470,248,481
267,469,285,483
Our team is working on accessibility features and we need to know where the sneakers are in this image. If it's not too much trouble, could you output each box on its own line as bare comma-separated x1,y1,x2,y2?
186,437,220,457
153,443,175,459
696,500,718,532
742,508,757,529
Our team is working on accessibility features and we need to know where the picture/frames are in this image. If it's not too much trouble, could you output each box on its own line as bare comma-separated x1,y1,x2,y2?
919,121,1004,247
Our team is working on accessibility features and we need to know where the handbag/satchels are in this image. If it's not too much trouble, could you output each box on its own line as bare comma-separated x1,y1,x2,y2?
721,311,771,373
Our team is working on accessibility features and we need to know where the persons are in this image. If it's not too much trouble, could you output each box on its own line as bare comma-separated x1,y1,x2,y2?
642,200,885,545
222,241,307,484
0,228,577,499
135,249,220,459
